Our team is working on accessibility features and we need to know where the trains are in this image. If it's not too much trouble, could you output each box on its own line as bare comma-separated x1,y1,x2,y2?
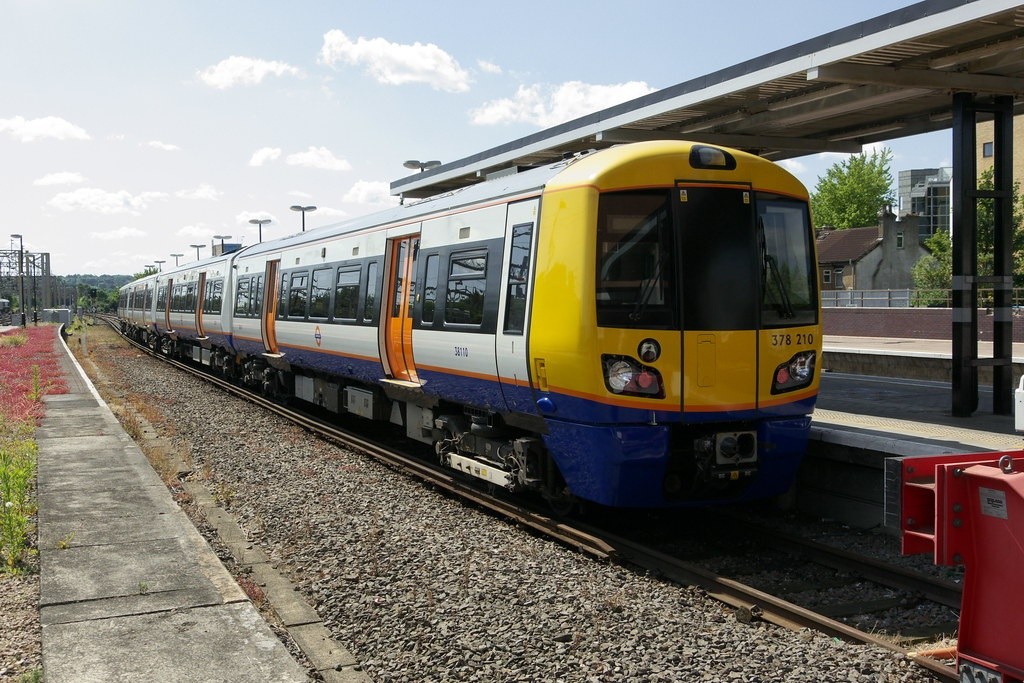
117,140,823,528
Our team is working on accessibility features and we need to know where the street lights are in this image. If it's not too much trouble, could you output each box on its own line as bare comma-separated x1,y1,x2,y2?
403,159,441,173
213,235,232,253
10,234,26,329
24,254,38,326
249,218,271,243
190,244,206,260
290,205,317,232
170,253,184,266
144,264,155,275
154,261,166,272
